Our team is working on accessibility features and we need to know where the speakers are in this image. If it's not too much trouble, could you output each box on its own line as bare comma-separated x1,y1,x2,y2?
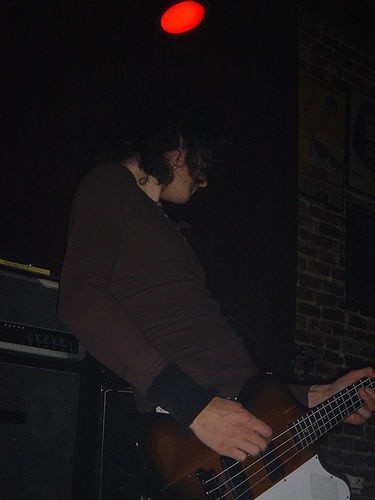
71,381,175,500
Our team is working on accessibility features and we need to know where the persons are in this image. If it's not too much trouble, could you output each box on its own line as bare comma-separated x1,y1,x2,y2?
57,91,375,499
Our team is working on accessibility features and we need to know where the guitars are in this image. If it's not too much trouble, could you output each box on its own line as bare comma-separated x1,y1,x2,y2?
106,372,374,500
